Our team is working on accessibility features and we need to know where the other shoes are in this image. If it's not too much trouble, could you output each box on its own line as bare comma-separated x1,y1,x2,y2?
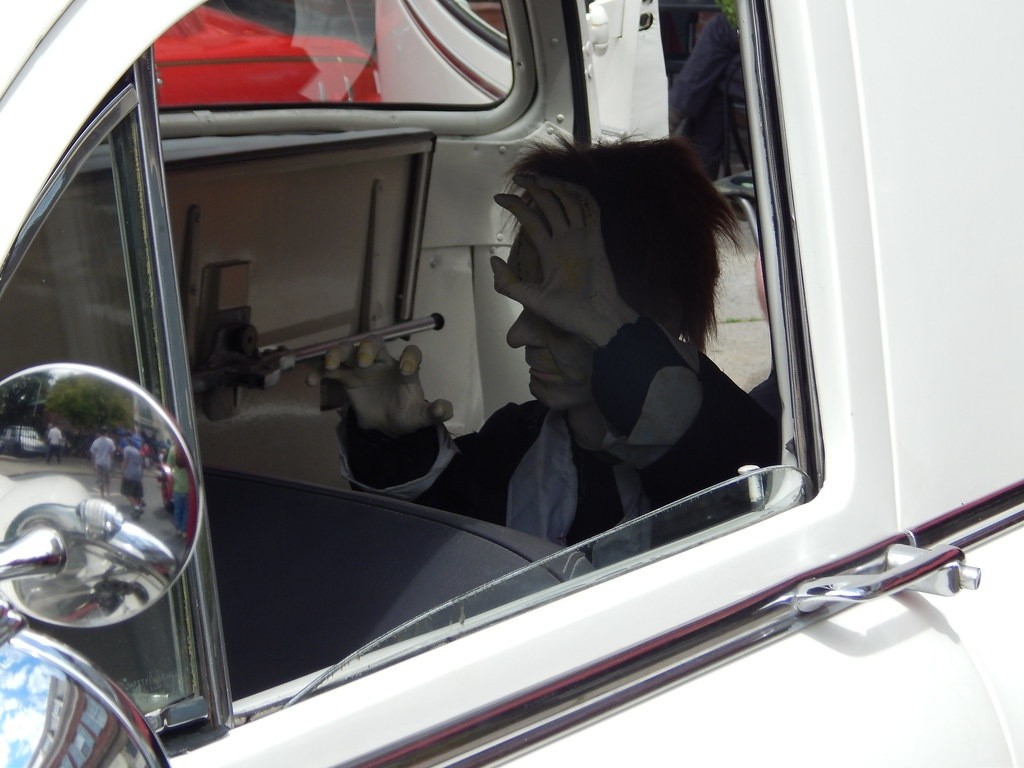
131,508,140,519
182,532,186,539
138,499,146,509
177,529,183,536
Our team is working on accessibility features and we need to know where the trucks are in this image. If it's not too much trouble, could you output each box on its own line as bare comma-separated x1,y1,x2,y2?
0,0,1024,768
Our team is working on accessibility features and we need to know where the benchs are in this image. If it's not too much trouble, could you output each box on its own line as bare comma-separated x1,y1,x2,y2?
201,462,594,691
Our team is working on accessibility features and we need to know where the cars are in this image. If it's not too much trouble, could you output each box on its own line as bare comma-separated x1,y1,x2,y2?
5,424,47,456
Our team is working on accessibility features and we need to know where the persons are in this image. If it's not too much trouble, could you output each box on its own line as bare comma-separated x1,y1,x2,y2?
41,421,197,541
306,130,784,574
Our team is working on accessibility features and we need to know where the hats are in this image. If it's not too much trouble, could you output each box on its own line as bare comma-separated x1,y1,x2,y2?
99,426,108,434
